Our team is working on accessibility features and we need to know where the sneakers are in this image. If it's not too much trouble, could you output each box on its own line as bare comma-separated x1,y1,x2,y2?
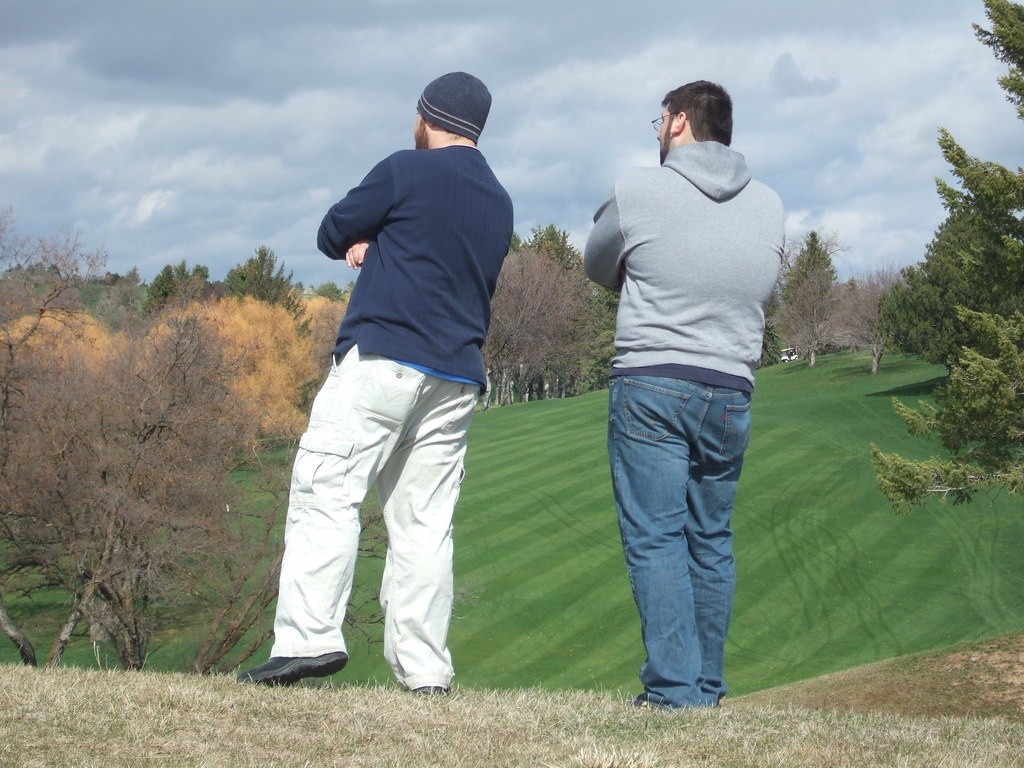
236,651,351,686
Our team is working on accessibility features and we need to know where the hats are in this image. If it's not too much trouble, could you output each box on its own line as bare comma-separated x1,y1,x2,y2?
417,71,492,143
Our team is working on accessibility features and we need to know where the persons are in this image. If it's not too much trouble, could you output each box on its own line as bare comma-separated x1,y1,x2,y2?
583,80,785,709
787,349,793,359
237,71,514,696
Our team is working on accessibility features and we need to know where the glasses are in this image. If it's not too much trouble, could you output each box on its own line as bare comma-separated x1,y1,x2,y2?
652,112,677,133
